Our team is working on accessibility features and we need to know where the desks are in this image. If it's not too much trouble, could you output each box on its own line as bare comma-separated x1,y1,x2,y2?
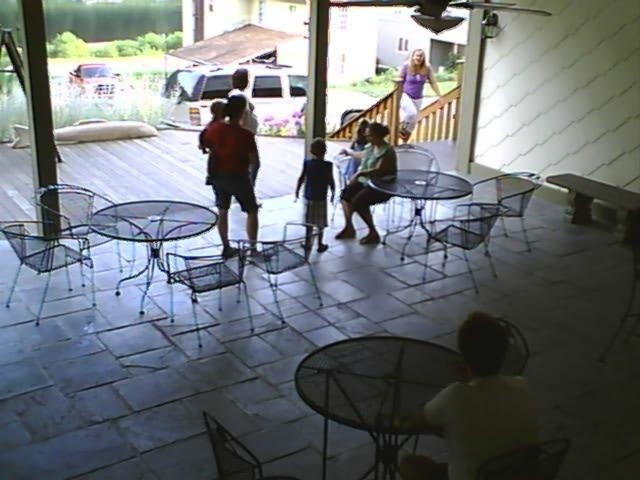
295,336,469,477
365,168,473,262
89,200,221,317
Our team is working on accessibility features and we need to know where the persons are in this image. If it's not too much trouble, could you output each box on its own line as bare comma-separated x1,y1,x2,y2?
201,100,228,185
213,95,259,257
399,312,544,479
399,49,442,142
335,121,396,245
295,137,335,252
228,68,263,208
345,118,372,180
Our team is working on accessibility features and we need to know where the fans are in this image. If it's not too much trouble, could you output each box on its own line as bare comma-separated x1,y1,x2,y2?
328,0,553,37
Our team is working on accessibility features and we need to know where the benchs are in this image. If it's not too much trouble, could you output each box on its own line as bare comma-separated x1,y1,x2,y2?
547,173,640,244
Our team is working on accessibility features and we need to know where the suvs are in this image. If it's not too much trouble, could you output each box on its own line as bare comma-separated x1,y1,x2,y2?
170,67,379,138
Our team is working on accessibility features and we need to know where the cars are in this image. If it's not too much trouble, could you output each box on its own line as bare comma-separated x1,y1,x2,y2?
69,64,125,99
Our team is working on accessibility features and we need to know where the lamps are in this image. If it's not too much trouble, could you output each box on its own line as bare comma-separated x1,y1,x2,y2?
480,13,500,38
412,14,464,35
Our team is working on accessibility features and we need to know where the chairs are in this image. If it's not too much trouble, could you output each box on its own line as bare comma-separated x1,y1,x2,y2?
167,241,256,349
202,411,265,479
429,203,510,295
33,185,125,292
468,172,543,256
1,223,97,327
329,156,373,226
390,143,440,231
468,437,573,478
492,317,530,374
228,221,323,323
